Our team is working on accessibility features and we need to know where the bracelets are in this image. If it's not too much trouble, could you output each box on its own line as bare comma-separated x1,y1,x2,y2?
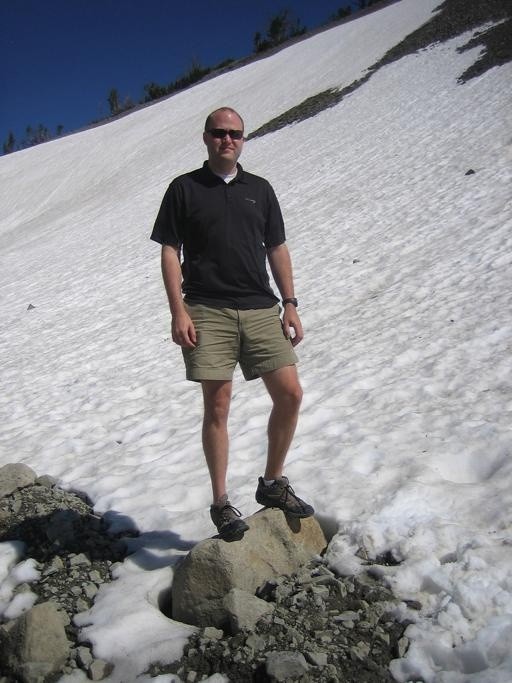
279,298,301,308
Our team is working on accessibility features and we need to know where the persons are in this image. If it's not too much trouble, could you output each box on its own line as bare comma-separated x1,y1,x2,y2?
148,108,316,537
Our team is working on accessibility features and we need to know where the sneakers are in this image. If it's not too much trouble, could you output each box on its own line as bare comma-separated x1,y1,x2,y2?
256,477,314,518
210,501,249,542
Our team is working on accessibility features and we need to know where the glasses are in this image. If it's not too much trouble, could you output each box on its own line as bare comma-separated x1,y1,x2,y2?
206,128,242,140
